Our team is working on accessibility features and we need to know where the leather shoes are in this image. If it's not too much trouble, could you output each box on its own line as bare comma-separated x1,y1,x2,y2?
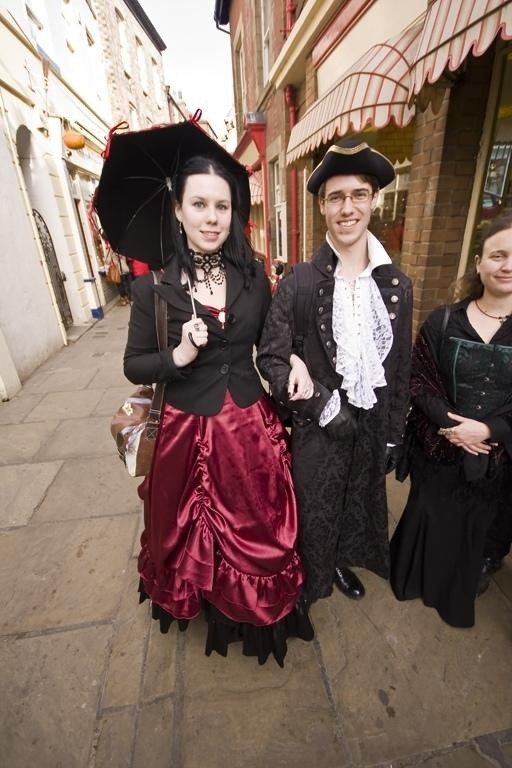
481,556,503,576
336,567,364,600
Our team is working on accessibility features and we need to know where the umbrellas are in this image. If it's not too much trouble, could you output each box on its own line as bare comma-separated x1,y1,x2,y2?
87,112,252,347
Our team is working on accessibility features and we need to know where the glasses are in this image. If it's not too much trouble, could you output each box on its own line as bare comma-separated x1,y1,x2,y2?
319,191,378,204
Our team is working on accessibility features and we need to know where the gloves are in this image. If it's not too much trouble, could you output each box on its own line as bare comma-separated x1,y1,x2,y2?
325,406,361,441
385,446,403,474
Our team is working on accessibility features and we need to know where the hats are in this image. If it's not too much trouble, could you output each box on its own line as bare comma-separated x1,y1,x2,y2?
306,135,396,194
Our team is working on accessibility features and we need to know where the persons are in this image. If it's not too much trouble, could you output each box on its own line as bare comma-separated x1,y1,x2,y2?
122,157,315,671
105,245,150,306
391,209,511,628
257,143,412,608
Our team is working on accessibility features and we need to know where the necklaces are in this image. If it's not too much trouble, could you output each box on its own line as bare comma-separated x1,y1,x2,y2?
184,247,227,294
475,299,510,326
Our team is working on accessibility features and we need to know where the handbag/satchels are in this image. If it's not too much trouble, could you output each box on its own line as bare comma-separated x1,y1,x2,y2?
111,384,157,477
396,405,419,482
105,263,121,284
